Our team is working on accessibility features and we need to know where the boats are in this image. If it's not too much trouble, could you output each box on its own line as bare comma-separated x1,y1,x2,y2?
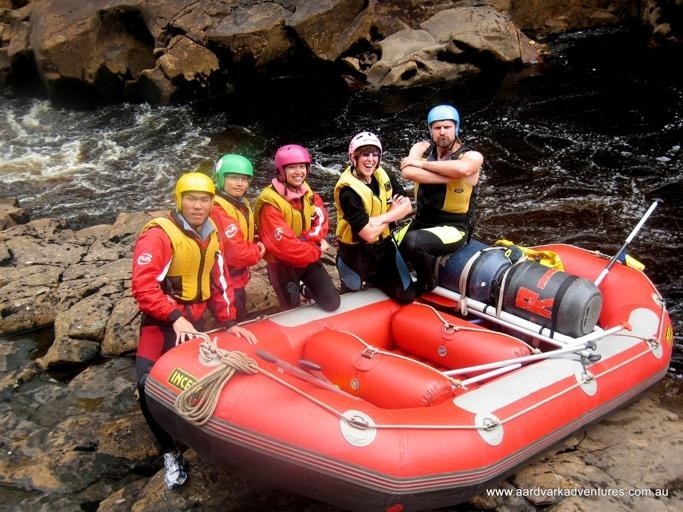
144,244,674,509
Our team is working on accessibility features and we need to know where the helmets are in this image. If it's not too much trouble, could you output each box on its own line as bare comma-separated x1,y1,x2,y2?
348,132,383,168
174,173,215,217
427,105,459,139
214,154,253,192
273,144,311,182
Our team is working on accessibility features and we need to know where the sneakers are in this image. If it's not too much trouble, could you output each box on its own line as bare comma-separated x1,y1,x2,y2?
161,445,187,491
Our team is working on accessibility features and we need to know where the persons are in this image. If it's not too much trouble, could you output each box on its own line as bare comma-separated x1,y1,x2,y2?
333,130,416,305
130,172,258,490
396,104,484,296
253,144,342,312
210,152,267,327
